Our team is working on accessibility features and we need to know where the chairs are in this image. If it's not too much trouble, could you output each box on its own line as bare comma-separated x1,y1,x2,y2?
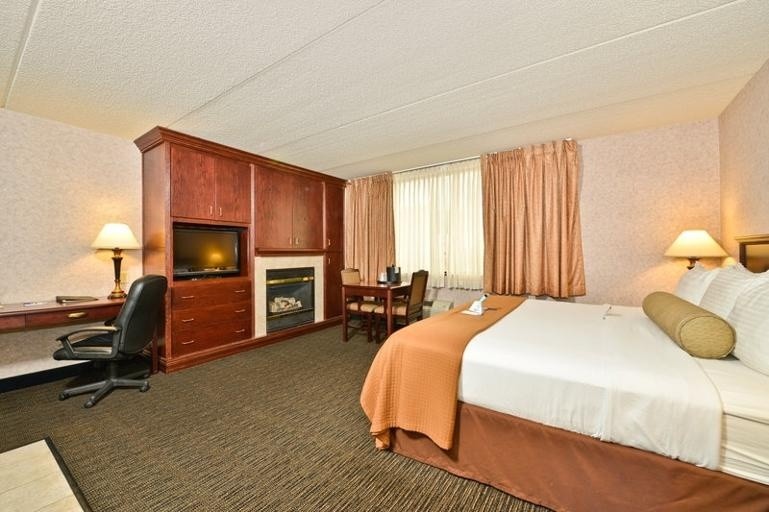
52,274,168,409
340,266,429,348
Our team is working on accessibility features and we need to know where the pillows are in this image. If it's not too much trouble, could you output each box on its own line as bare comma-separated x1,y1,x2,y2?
642,256,768,377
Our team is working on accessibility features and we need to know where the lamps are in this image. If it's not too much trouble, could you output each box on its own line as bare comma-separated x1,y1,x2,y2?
91,223,142,299
663,229,730,271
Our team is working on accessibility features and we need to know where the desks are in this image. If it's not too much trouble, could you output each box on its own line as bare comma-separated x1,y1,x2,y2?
0,296,158,375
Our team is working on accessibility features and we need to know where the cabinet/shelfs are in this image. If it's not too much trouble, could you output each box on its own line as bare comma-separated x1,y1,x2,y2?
133,125,348,375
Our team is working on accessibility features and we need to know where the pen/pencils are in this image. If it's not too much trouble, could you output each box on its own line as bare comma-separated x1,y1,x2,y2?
24,301,48,306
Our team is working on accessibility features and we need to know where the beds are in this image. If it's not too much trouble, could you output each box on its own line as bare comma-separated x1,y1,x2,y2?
361,234,768,511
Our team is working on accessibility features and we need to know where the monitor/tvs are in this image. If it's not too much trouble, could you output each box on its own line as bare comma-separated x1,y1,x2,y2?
173,225,242,281
